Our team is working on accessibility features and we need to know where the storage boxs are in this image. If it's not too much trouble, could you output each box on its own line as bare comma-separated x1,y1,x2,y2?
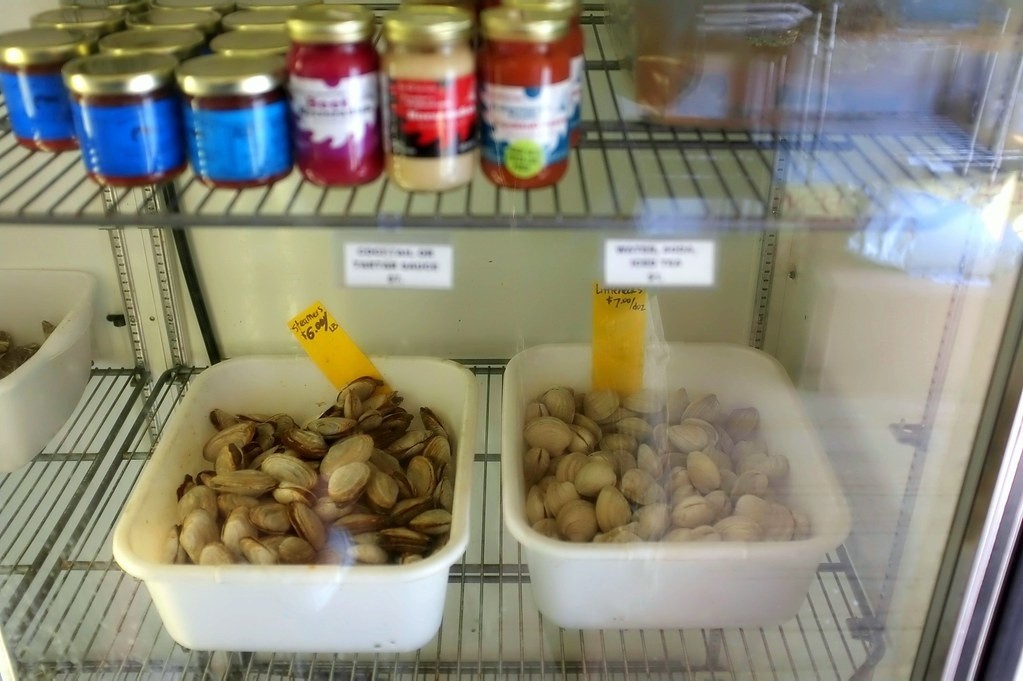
500,342,847,629
0,265,101,472
112,353,476,654
